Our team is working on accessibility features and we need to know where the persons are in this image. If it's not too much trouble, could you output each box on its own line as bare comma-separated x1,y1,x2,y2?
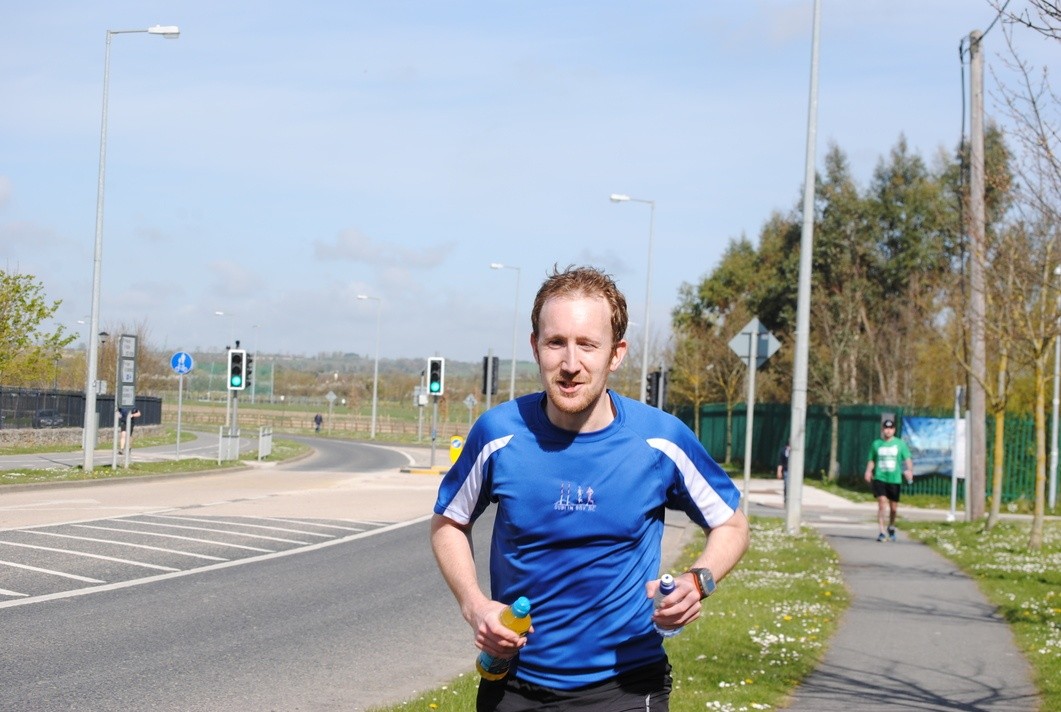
776,437,790,507
431,264,751,712
313,412,323,434
119,406,141,454
866,419,913,542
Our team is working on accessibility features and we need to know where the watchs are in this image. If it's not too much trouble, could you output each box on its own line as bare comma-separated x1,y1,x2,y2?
684,568,717,600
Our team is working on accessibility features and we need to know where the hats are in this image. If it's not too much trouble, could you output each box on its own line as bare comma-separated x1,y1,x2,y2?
883,420,894,428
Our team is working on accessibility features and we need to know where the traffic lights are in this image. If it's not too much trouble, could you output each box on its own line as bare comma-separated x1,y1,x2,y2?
427,358,445,396
245,353,253,388
646,371,659,407
227,349,246,390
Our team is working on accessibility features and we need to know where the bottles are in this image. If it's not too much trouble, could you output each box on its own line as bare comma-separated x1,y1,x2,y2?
653,573,684,636
475,596,534,680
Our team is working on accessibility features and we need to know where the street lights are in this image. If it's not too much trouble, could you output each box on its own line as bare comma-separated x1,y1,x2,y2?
214,310,227,353
97,331,109,397
491,263,521,401
610,193,656,404
356,294,383,439
83,23,182,472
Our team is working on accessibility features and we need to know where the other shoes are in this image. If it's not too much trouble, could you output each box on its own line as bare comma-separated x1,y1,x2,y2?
887,527,896,542
877,533,885,542
